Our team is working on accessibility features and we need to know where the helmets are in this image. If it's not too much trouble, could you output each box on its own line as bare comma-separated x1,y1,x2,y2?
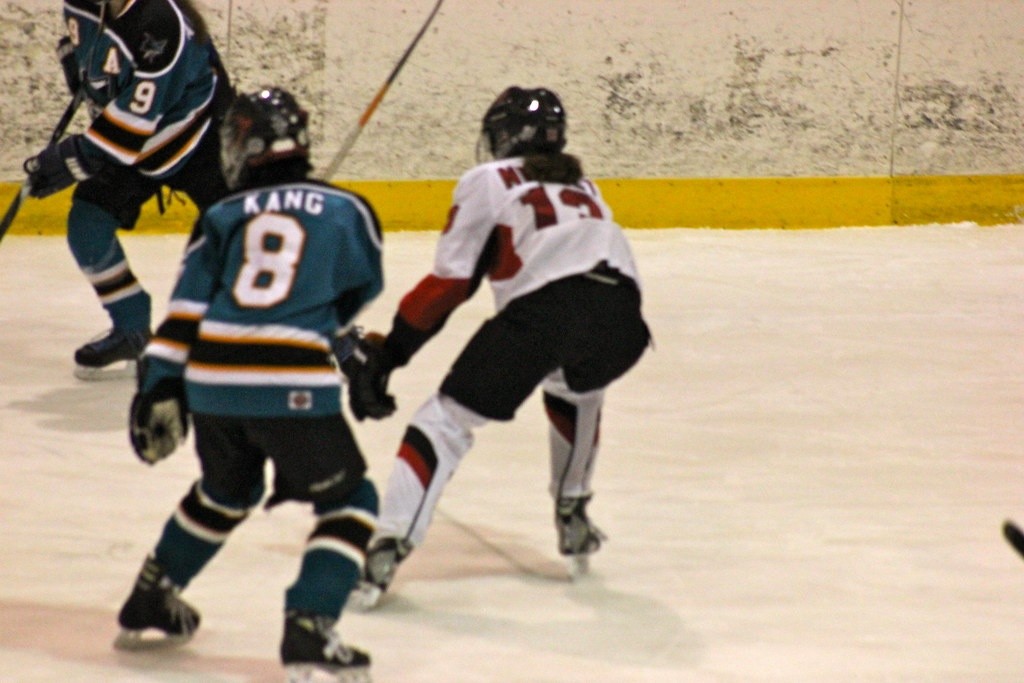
481,86,562,160
221,84,314,191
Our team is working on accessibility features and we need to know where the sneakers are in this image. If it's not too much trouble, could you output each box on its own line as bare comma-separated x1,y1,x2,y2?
357,540,411,591
74,324,157,368
553,490,605,557
280,604,370,672
118,557,205,637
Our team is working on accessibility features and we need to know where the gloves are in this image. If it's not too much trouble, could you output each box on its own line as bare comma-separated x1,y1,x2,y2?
333,333,396,418
130,376,188,463
22,135,114,200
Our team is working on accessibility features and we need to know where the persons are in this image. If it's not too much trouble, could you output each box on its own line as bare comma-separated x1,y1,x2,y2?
24,1,240,370
361,87,651,591
117,86,386,671
